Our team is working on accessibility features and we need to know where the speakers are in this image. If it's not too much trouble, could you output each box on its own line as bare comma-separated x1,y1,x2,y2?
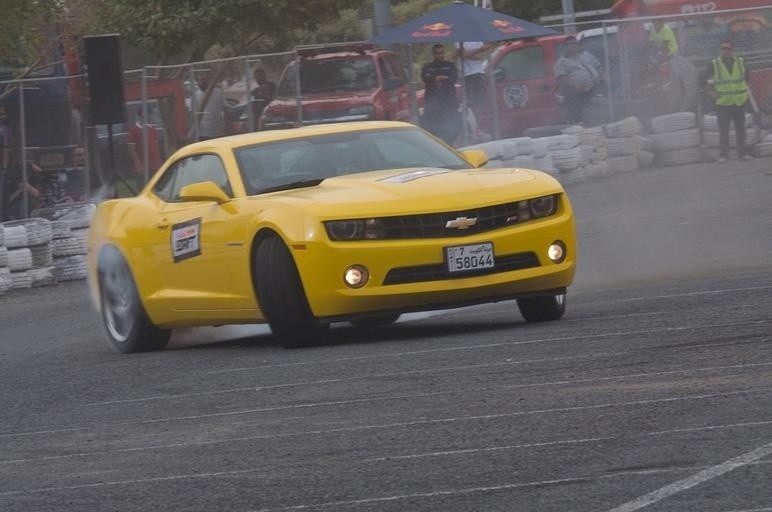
82,33,128,125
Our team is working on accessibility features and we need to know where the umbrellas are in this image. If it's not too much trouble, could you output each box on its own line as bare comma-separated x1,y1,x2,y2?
361,1,561,47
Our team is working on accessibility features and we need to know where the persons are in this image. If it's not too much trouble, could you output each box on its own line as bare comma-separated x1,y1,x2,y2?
190,73,231,140
252,68,276,115
455,40,492,108
648,21,678,57
0,104,15,192
420,43,461,137
71,147,104,198
552,36,603,117
126,105,162,190
700,39,756,164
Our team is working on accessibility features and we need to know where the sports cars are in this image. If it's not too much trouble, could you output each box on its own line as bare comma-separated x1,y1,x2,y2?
87,120,576,351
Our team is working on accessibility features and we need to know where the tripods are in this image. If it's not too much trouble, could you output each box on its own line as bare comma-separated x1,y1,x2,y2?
98,126,137,199
